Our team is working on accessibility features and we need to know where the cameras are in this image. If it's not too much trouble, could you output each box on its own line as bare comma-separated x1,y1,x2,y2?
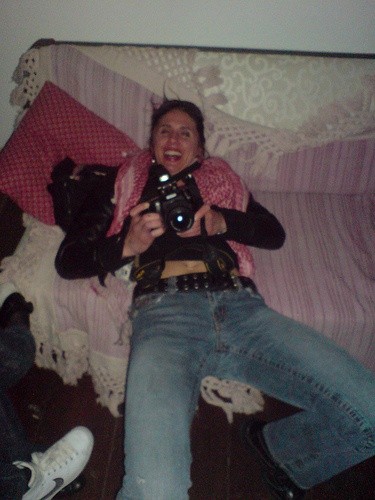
138,162,204,233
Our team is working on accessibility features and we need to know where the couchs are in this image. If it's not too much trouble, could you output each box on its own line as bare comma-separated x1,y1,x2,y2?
0,38,375,424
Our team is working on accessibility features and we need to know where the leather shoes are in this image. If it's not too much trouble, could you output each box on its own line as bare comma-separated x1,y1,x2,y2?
240,421,307,500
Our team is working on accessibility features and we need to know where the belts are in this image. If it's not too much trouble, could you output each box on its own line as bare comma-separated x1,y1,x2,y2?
132,272,257,300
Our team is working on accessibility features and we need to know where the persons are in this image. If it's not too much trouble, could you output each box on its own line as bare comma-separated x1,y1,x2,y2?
0,424,95,499
53,98,375,500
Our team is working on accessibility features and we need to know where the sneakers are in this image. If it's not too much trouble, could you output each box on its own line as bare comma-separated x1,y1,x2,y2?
15,426,94,500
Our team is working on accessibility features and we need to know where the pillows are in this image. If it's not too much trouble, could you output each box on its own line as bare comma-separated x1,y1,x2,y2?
1,80,146,227
